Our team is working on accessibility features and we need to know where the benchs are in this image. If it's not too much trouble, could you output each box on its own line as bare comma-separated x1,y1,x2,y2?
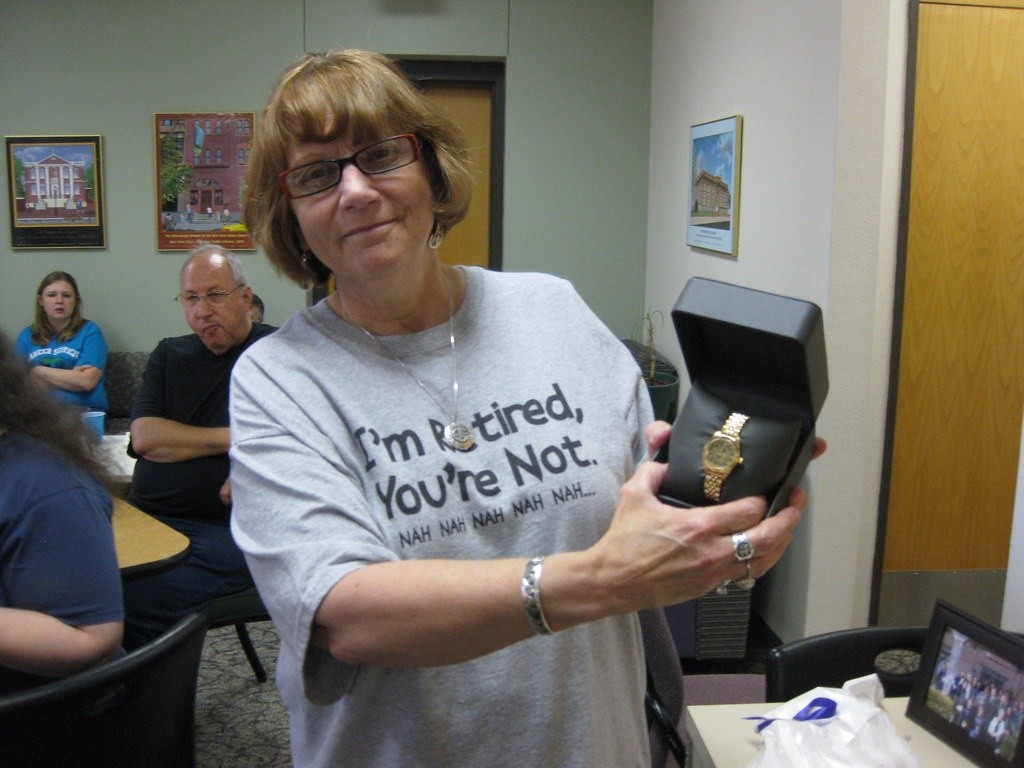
102,351,152,500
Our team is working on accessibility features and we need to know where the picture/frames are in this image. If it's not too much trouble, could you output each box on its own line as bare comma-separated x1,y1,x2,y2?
685,114,743,257
152,112,257,252
4,134,106,250
906,597,1024,768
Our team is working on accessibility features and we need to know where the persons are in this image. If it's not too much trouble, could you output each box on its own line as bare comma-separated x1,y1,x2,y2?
13,271,109,434
121,242,279,768
226,51,829,767
934,662,1024,754
0,329,131,698
249,293,265,323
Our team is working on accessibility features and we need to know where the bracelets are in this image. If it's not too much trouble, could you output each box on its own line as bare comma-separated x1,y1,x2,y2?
517,556,561,639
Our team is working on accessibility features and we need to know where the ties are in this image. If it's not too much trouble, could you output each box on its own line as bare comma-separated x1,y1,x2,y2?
992,720,1002,740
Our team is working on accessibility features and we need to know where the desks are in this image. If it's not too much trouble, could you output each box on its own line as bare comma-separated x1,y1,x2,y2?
110,494,192,584
685,697,981,767
81,434,138,483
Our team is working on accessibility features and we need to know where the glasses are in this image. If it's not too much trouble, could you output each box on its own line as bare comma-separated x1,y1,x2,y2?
174,284,244,307
278,133,426,201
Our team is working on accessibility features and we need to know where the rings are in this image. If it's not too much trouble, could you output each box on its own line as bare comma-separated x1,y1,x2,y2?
717,580,732,595
731,532,754,562
736,562,755,591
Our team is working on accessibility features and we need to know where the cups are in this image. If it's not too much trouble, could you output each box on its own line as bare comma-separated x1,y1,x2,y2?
81,411,106,446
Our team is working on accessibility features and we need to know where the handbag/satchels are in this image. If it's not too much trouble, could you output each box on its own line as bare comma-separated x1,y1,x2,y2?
740,672,919,768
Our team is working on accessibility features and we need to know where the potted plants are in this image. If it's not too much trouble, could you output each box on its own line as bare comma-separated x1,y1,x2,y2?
630,311,679,422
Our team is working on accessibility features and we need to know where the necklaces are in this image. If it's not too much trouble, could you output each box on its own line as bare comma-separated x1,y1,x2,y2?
336,267,475,452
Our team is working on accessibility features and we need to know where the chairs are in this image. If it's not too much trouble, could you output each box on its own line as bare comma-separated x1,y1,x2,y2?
637,605,768,768
764,625,1024,703
0,588,273,768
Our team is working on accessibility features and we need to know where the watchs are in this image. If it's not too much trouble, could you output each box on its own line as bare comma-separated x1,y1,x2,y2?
702,410,752,503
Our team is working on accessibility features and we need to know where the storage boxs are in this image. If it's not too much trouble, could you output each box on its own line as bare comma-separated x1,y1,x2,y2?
651,275,829,525
